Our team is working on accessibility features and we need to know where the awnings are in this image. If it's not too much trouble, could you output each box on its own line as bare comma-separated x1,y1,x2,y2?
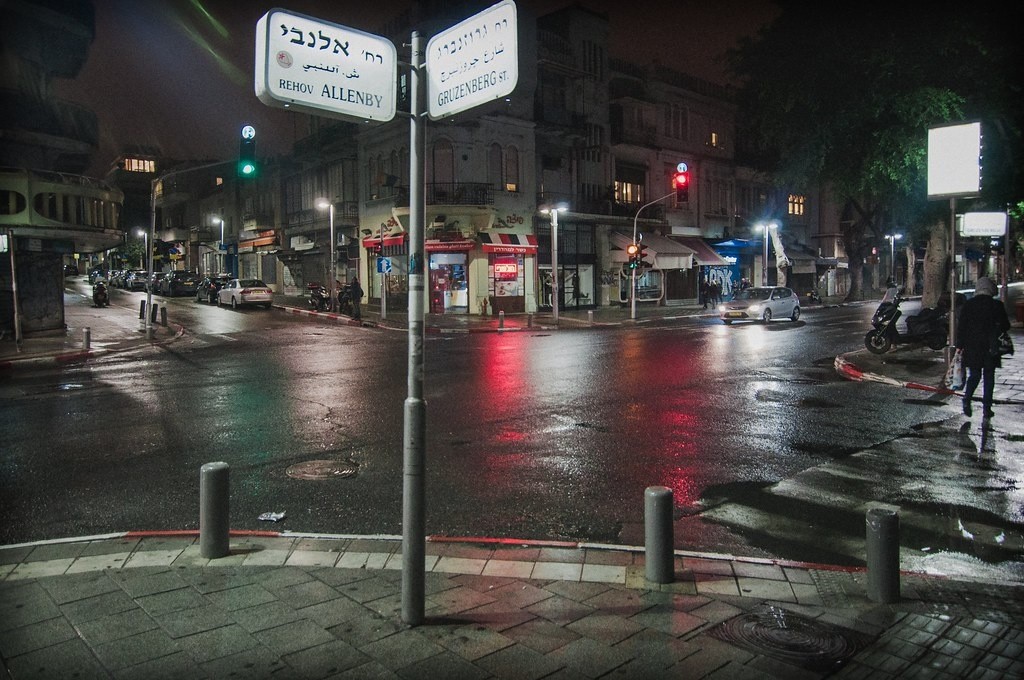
479,233,540,255
362,231,408,247
610,229,693,271
237,236,278,254
666,234,731,266
426,237,476,251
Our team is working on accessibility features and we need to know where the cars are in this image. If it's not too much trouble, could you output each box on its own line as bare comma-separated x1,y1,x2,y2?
64,264,273,310
718,286,802,323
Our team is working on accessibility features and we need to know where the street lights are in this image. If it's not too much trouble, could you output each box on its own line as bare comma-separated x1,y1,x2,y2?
754,224,779,285
538,202,570,322
318,201,337,310
138,231,148,270
212,217,224,274
883,233,903,288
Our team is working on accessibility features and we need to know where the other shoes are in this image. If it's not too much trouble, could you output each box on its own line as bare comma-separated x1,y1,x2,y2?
983,405,994,416
962,398,972,417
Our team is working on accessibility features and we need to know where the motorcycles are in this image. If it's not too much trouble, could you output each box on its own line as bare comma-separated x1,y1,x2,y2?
335,279,355,316
807,286,822,304
307,283,332,312
865,288,967,354
92,282,110,308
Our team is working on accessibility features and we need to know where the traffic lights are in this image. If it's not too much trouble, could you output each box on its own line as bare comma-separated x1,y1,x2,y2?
675,173,689,202
631,256,635,269
642,244,649,266
627,245,637,256
240,138,256,177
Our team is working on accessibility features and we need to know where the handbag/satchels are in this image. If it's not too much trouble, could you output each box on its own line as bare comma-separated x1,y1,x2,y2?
944,350,966,390
991,331,1014,357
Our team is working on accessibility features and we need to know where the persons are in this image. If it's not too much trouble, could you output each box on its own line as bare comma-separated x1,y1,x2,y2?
701,278,753,309
955,276,1015,417
350,276,362,321
